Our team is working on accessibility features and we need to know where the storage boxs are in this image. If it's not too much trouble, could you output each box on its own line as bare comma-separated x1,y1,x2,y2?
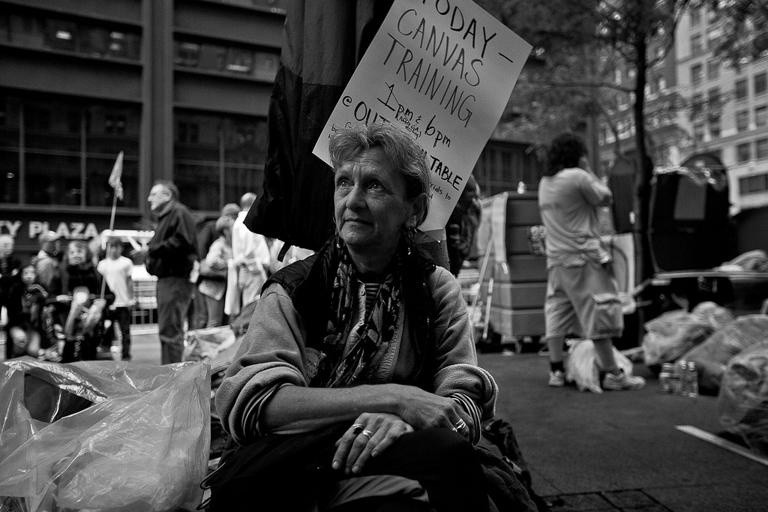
481,200,548,336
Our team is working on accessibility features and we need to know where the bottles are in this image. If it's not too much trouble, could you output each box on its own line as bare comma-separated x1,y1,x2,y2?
659,360,698,398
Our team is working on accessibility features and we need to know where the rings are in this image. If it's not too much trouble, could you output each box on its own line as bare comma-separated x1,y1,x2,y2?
456,418,467,430
350,423,363,434
360,430,373,439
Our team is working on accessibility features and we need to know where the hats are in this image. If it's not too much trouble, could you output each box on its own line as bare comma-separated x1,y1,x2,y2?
38,232,65,243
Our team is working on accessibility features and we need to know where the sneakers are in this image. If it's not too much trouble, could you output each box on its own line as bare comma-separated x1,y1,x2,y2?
604,371,646,392
548,368,565,386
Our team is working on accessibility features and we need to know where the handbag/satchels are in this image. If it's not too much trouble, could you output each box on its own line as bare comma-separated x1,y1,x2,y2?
200,260,227,281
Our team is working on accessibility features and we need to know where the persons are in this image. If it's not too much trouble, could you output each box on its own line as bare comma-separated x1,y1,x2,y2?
191,204,237,330
538,132,646,391
0,232,134,364
232,192,269,324
198,216,243,327
128,182,198,365
215,122,500,511
